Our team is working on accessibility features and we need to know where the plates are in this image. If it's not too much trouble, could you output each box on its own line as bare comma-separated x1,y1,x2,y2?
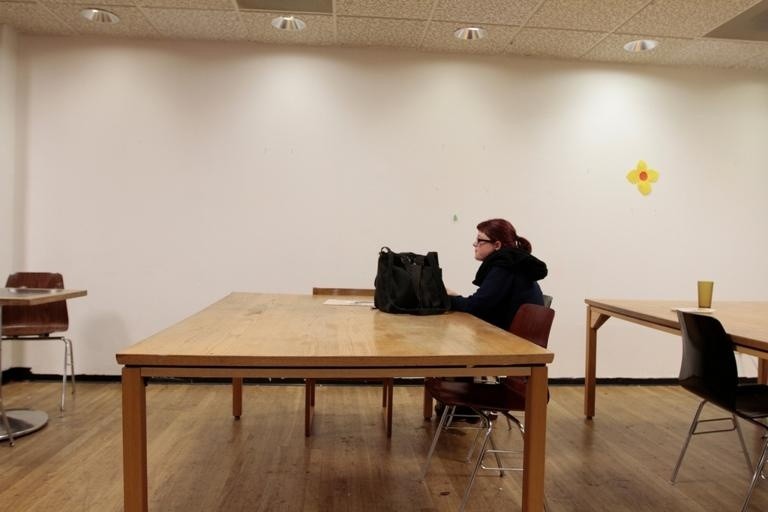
674,306,714,314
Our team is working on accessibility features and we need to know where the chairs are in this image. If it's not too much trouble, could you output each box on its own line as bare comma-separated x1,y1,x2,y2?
672,306,768,511
3,271,79,414
423,301,555,508
304,287,395,440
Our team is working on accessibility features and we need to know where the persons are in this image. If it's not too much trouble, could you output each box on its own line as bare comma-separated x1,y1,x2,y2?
434,219,548,425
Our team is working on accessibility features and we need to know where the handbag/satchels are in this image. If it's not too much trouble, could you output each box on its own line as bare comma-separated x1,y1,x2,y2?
372,246,451,315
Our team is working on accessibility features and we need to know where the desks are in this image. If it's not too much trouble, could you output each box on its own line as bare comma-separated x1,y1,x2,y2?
582,292,768,429
2,286,87,443
115,292,554,511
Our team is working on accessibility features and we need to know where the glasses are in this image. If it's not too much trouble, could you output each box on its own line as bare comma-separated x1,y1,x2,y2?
477,239,493,243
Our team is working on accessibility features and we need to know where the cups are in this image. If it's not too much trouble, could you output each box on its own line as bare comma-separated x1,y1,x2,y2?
697,280,715,308
